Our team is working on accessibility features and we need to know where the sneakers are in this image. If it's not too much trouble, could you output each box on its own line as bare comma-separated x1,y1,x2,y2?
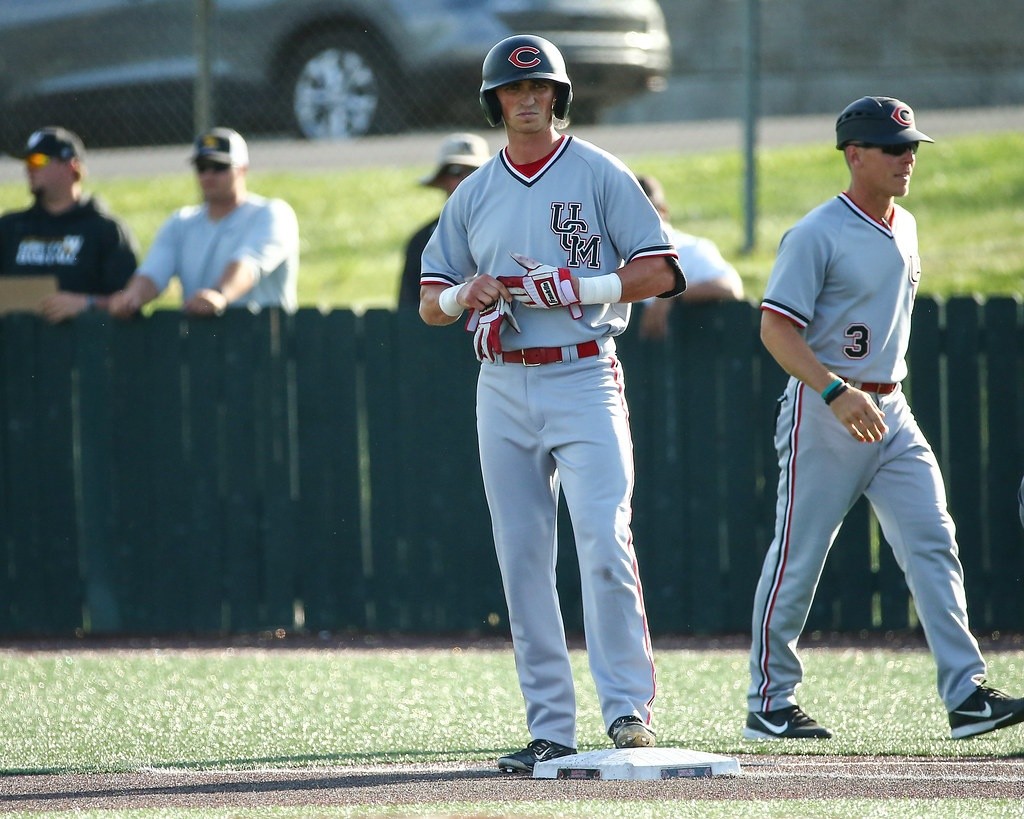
611,716,656,748
948,681,1024,739
498,738,578,773
743,705,833,742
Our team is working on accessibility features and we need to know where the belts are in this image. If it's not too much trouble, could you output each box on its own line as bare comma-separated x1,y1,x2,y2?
837,376,897,395
484,341,599,367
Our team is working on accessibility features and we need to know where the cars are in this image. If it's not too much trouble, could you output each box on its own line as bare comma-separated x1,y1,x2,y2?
0,1,674,139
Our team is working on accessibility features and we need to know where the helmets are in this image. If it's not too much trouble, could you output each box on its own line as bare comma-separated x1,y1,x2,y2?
424,130,491,185
478,34,573,128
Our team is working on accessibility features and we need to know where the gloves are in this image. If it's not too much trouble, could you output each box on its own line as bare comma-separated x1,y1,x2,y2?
495,251,584,319
466,293,522,363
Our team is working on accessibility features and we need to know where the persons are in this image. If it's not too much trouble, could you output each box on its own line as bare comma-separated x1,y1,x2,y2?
742,93,1024,737
420,33,687,772
398,133,496,315
109,127,300,317
635,175,743,335
0,124,138,322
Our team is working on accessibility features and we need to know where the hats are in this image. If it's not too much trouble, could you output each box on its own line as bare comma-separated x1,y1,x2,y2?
834,96,933,151
18,125,86,160
188,128,249,164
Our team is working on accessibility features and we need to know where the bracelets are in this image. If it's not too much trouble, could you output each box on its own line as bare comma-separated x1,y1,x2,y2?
826,383,850,406
578,273,622,305
438,282,468,317
821,379,844,400
85,294,97,313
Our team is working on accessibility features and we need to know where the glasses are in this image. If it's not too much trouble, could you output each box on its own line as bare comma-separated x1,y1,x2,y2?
443,164,479,177
24,152,51,168
196,159,231,172
849,141,920,156
